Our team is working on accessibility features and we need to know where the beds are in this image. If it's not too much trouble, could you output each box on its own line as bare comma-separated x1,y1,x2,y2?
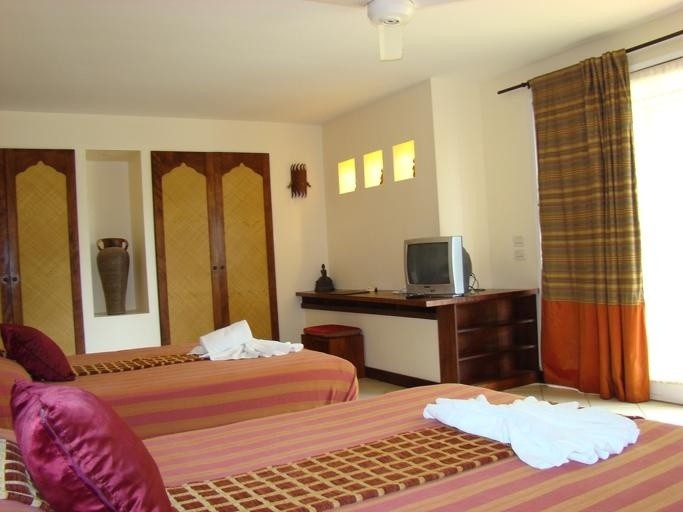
0,319,360,442
0,383,683,512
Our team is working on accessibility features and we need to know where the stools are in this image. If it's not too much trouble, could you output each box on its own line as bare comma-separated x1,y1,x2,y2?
300,323,366,377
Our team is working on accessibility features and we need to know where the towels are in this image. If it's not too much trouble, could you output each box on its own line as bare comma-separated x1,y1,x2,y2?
187,319,305,361
422,395,640,469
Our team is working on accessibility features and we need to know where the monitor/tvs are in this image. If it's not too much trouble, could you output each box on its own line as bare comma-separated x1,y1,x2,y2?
404,235,472,297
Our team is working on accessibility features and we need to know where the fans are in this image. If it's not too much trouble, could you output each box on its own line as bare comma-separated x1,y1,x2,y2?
311,0,463,61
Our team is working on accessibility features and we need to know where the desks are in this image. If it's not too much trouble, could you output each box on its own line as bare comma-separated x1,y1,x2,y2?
296,287,538,392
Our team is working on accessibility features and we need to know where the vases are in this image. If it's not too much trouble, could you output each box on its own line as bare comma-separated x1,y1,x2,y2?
97,236,130,315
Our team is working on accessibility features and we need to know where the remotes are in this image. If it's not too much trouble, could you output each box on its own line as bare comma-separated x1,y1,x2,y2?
406,294,424,299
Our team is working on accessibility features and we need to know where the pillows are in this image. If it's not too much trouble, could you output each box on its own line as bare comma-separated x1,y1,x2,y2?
10,379,173,512
0,324,75,381
